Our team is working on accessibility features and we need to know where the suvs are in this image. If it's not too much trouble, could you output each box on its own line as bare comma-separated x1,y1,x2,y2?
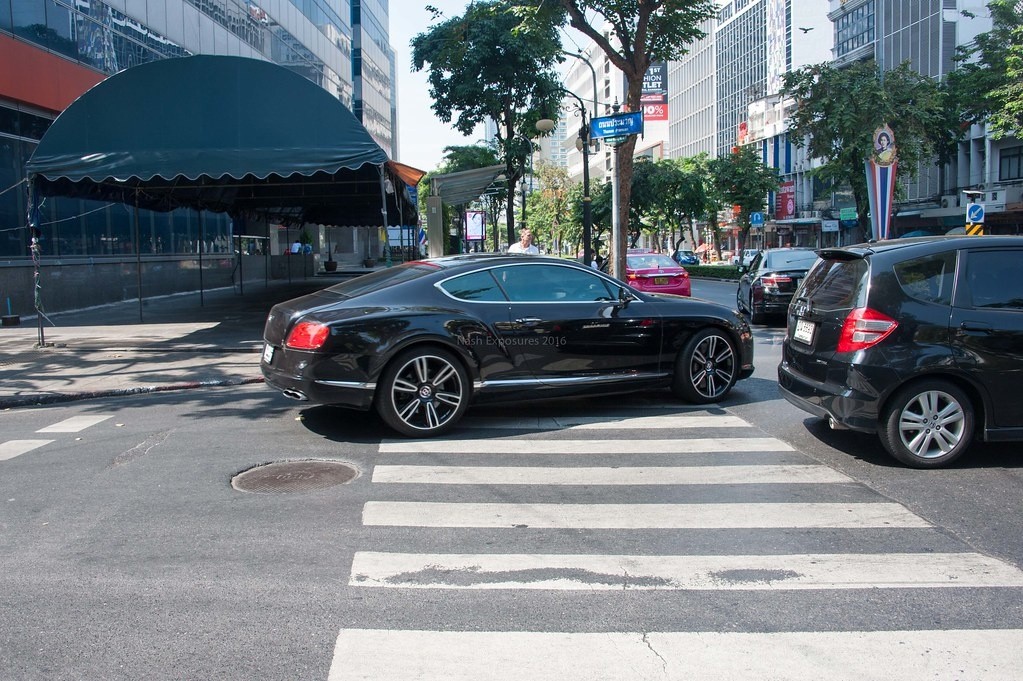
777,235,1022,470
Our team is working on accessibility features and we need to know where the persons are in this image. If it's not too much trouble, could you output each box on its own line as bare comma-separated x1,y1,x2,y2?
877,132,892,160
786,240,791,246
508,230,539,255
591,249,603,270
291,240,312,255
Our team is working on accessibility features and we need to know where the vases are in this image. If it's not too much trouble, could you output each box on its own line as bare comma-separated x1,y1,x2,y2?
324,261,337,271
364,259,374,268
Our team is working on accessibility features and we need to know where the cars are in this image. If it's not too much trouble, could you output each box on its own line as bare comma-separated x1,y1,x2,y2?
598,247,692,297
735,247,825,326
671,250,700,267
259,251,756,440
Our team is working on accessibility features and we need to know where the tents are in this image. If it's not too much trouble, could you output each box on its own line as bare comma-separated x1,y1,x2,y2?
27,53,427,345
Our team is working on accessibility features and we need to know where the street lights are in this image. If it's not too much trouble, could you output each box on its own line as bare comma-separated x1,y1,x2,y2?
535,88,592,268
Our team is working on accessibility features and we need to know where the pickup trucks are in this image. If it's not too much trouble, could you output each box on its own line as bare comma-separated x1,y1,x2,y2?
730,248,762,268
